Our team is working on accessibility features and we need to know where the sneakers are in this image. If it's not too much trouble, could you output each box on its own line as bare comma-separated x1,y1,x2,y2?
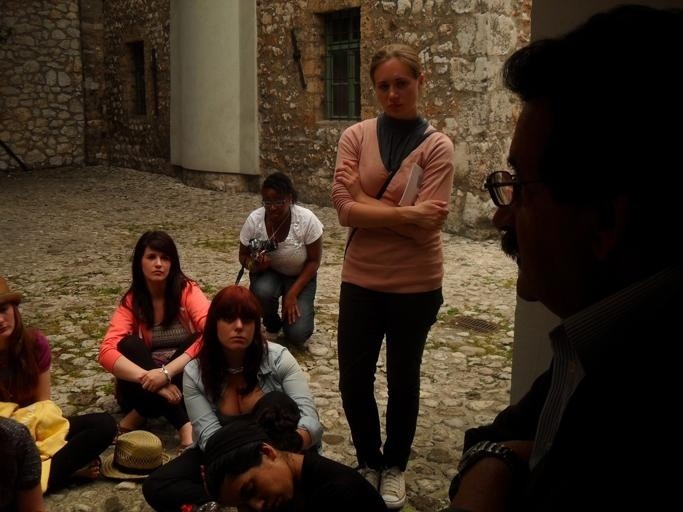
356,461,379,490
265,329,280,340
380,463,406,509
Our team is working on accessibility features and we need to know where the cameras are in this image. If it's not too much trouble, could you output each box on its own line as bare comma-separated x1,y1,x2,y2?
248,237,278,259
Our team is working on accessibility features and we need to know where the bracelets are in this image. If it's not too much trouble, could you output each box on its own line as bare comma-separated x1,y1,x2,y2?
160,360,173,386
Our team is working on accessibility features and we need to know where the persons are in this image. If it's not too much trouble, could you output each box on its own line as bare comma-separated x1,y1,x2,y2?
330,40,453,509
141,285,323,512
0,276,114,498
0,418,51,511
235,170,327,344
437,0,683,510
95,230,212,454
200,401,388,511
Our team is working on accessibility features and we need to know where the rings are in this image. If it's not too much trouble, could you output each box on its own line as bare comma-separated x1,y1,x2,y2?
175,393,179,399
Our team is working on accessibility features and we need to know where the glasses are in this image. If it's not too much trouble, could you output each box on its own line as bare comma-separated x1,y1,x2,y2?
485,171,522,207
261,198,290,207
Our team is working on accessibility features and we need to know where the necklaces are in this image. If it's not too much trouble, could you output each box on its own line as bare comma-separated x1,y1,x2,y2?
224,361,250,374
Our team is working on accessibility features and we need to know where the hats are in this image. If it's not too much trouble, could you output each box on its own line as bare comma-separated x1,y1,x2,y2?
100,430,170,479
0,276,21,306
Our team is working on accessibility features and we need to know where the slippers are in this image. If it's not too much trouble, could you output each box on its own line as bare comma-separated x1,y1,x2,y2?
73,456,101,484
111,422,131,445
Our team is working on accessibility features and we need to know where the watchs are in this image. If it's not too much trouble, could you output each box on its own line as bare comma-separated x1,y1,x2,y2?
447,437,516,490
